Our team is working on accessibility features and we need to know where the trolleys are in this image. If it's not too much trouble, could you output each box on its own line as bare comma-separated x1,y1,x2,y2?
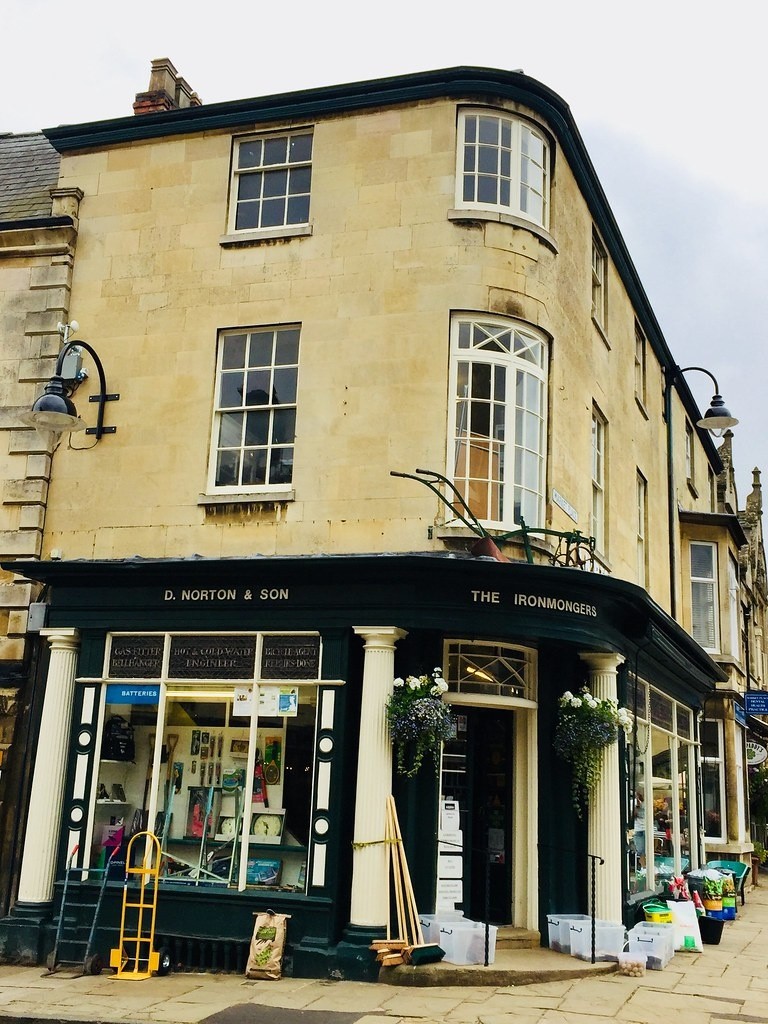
40,843,123,980
109,831,174,980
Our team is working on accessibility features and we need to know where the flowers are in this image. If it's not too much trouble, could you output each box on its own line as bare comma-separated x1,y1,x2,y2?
551,680,633,823
383,666,458,779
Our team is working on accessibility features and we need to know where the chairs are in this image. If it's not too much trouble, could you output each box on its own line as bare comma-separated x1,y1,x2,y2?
707,860,751,906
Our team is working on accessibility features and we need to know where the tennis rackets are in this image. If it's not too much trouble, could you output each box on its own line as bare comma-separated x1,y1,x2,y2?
264,741,280,785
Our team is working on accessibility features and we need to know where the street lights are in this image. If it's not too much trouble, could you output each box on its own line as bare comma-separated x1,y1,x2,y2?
664,365,740,620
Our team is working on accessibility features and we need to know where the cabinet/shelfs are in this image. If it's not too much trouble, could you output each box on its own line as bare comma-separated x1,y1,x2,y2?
95,759,136,805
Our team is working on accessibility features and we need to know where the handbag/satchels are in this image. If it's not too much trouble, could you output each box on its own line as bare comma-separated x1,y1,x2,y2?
102,714,137,762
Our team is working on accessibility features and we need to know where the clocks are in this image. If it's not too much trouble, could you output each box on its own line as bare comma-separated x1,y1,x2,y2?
251,814,282,837
219,816,243,835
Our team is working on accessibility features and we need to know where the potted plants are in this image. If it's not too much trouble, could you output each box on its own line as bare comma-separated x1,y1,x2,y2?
750,841,768,886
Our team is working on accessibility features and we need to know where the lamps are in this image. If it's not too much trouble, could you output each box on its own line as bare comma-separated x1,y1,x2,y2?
17,338,121,440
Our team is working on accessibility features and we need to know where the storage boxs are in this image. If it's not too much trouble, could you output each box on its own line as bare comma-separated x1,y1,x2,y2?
101,825,126,846
236,858,284,886
547,914,594,955
96,845,136,880
643,903,672,923
617,951,648,977
569,920,626,963
628,927,674,971
419,912,474,944
634,921,678,960
183,786,223,840
439,921,498,966
